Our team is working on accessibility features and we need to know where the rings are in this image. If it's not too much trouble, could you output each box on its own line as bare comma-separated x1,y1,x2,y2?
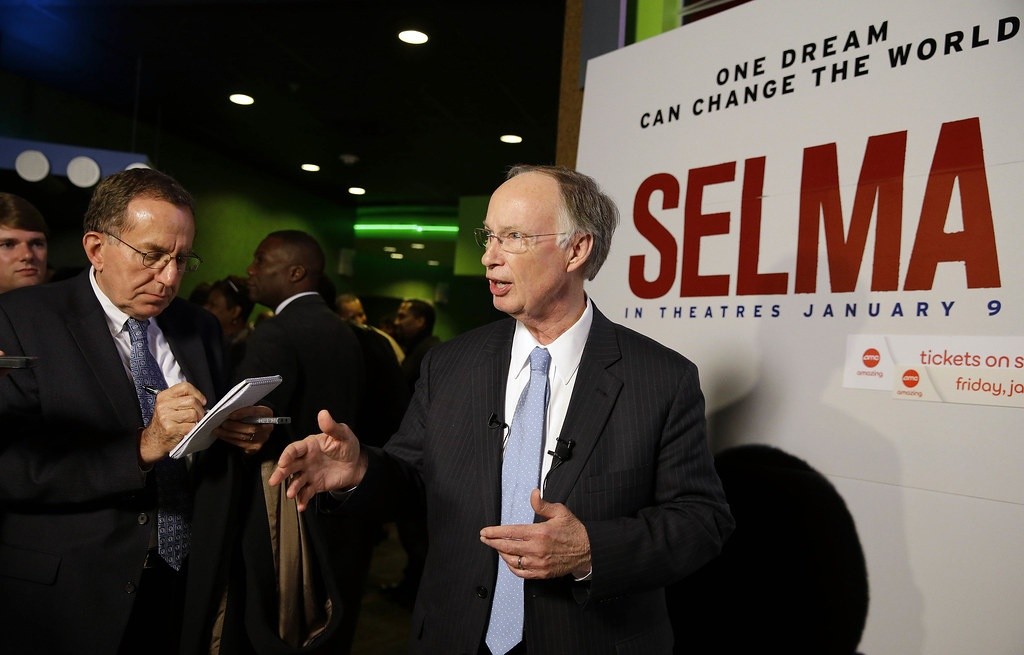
517,556,522,569
249,434,255,441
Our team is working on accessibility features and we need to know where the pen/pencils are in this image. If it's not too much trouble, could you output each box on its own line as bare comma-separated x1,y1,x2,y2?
142,385,210,413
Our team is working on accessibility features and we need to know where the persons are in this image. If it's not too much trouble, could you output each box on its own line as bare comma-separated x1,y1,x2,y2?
0,168,273,655
191,167,734,655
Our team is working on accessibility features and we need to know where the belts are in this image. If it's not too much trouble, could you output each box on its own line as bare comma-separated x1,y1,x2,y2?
141,552,190,573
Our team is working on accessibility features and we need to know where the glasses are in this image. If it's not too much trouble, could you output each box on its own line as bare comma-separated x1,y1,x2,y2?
474,227,580,254
225,276,245,295
101,229,202,274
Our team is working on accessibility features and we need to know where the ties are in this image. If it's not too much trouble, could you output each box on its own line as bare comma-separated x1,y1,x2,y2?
485,346,552,655
124,316,192,572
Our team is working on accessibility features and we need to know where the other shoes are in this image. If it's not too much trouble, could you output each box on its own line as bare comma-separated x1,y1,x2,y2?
382,586,414,612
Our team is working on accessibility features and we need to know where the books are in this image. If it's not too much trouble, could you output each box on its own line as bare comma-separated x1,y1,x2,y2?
168,375,282,459
